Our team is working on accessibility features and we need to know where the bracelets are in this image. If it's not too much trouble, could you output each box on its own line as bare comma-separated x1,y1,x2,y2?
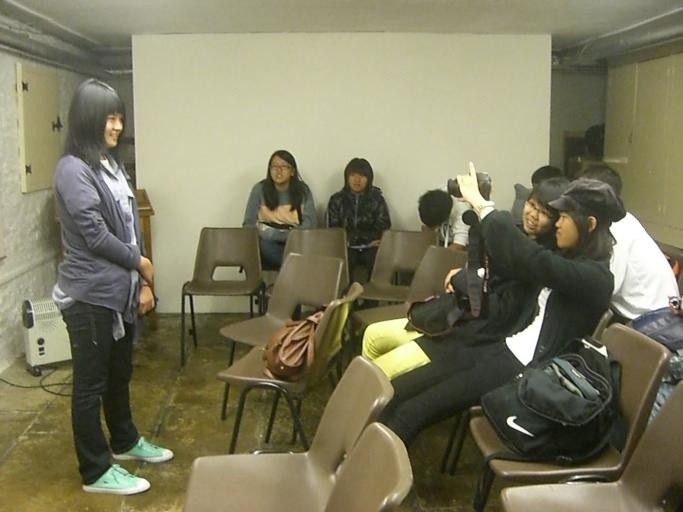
142,284,152,287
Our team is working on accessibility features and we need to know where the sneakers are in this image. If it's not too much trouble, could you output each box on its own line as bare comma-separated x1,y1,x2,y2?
80,461,150,497
109,435,175,464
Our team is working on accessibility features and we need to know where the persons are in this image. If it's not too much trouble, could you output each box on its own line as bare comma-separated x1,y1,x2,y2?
362,170,570,381
375,162,626,444
418,189,478,250
531,162,683,429
324,158,391,311
245,149,316,321
51,76,175,496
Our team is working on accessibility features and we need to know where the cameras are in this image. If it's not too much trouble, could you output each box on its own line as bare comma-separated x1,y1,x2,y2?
448,173,492,203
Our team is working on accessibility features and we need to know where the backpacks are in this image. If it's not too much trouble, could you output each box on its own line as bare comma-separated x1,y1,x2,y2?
479,334,626,466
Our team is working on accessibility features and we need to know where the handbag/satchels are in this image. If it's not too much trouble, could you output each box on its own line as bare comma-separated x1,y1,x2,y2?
260,310,326,382
631,295,683,351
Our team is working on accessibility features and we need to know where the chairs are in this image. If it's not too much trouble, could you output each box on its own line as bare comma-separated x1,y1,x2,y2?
357,223,683,511
217,282,366,454
282,228,350,292
185,352,416,510
179,223,267,366
215,252,344,424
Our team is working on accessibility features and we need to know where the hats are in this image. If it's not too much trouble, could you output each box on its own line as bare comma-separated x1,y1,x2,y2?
545,176,627,224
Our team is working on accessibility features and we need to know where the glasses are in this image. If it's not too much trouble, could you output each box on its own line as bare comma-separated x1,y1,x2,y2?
523,198,556,222
269,163,292,171
